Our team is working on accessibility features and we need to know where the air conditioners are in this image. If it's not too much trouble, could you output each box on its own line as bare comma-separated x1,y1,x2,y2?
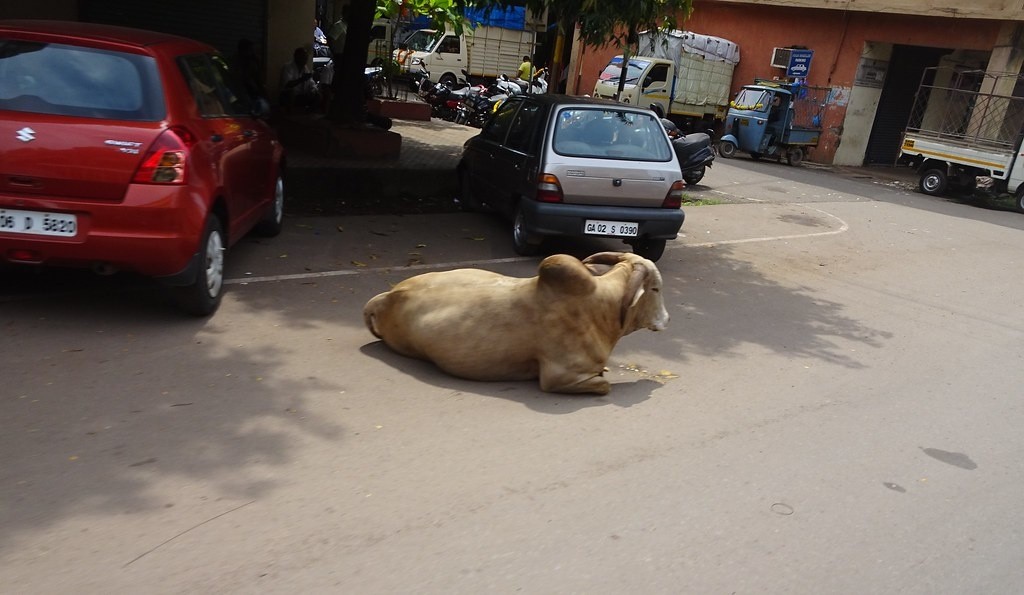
770,47,795,69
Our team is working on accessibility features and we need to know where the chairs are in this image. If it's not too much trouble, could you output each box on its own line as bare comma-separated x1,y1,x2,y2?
82,85,125,120
585,119,614,142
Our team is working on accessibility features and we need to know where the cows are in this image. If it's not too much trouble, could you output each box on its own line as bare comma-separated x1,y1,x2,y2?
363,250,671,397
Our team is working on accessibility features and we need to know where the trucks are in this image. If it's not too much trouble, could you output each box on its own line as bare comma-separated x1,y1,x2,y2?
593,55,728,140
894,66,1024,214
366,19,537,89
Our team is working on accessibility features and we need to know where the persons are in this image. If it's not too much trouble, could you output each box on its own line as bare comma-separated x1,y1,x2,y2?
512,55,537,83
280,47,318,106
223,38,272,115
559,63,569,85
312,18,327,44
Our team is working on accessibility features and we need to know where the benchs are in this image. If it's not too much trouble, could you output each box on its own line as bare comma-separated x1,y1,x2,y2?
557,141,646,157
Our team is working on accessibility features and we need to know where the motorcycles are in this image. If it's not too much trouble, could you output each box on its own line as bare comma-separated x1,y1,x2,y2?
719,78,830,167
312,24,336,58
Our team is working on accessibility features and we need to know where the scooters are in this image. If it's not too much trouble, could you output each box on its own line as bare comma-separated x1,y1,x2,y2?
650,102,717,185
412,60,549,126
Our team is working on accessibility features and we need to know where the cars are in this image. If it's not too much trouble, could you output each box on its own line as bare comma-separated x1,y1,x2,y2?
0,20,287,316
464,94,687,263
